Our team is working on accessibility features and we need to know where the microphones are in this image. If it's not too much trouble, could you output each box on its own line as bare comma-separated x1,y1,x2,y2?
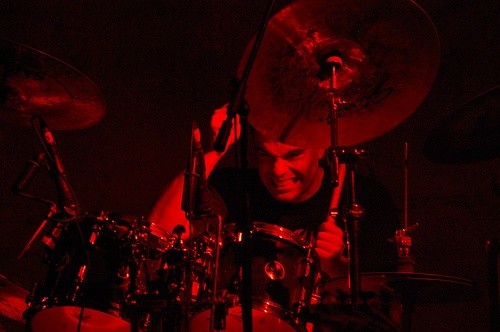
190,120,216,214
37,117,79,209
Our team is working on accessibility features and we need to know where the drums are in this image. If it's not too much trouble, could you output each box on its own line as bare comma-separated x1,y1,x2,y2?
110,232,206,308
50,260,110,310
0,273,35,330
207,219,313,310
21,295,133,331
190,300,313,332
57,212,134,259
313,273,406,329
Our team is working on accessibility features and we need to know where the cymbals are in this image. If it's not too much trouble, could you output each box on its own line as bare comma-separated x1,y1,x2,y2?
1,38,106,131
336,268,480,306
239,0,442,150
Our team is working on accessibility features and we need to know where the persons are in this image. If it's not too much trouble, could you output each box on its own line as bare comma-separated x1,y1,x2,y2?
179,104,410,332
